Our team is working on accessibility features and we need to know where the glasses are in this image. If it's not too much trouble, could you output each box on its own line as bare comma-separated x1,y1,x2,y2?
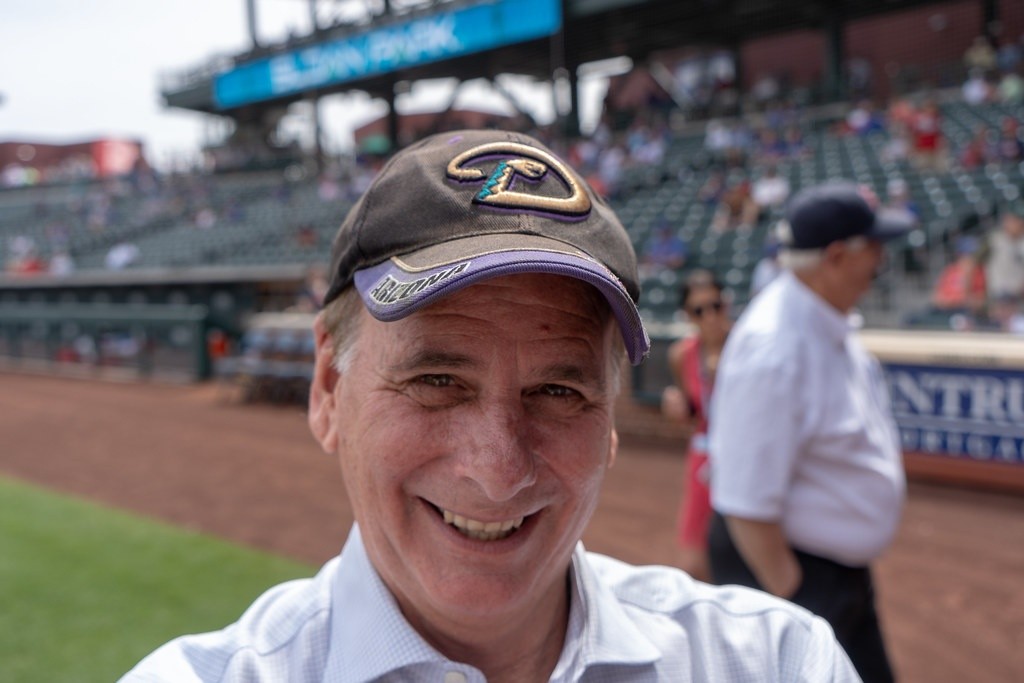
688,301,719,313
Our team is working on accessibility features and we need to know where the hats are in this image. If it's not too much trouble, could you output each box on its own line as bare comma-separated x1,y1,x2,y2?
773,183,916,253
324,129,653,365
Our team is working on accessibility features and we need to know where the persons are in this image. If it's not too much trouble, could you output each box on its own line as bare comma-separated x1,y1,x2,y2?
667,159,934,683
9,136,386,274
844,34,1024,171
936,215,1024,332
566,44,814,201
121,130,863,683
